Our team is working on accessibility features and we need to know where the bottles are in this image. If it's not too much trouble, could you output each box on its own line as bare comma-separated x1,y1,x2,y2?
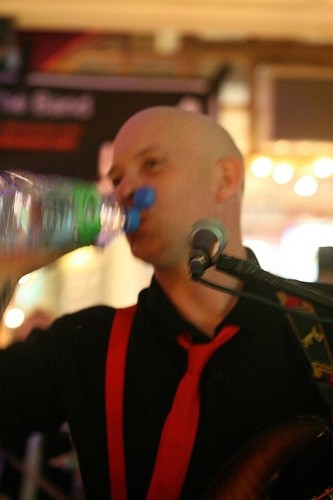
1,169,155,251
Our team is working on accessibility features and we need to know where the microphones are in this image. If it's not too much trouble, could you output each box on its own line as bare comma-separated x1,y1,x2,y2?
186,219,226,282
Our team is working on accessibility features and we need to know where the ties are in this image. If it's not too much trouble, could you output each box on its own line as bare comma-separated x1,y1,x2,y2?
145,325,243,500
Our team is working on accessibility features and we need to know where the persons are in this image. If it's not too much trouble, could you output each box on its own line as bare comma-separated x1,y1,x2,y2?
0,107,333,500
